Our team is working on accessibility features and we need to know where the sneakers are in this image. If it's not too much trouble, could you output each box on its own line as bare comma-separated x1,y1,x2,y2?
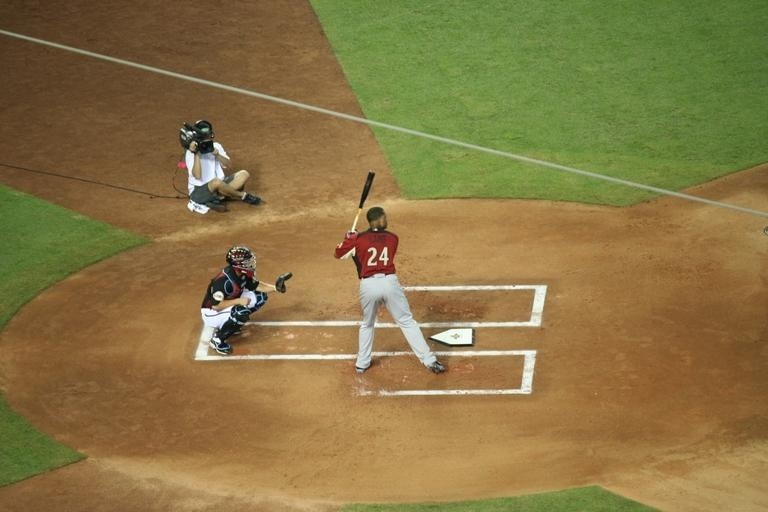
431,360,446,375
206,199,227,212
242,192,261,205
208,337,231,355
356,361,372,372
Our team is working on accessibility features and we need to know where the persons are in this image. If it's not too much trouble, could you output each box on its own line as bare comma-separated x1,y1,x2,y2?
201,246,293,356
333,208,445,374
181,120,261,213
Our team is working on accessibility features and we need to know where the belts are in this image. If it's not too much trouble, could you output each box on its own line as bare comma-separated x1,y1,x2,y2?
362,272,395,279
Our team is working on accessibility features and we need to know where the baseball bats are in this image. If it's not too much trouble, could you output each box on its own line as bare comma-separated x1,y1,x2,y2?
351,172,375,232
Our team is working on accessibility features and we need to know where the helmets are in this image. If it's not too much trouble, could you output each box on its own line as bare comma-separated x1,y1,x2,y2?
194,120,213,132
225,245,256,272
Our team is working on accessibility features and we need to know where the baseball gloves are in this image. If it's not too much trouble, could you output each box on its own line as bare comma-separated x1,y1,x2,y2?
276,273,293,293
